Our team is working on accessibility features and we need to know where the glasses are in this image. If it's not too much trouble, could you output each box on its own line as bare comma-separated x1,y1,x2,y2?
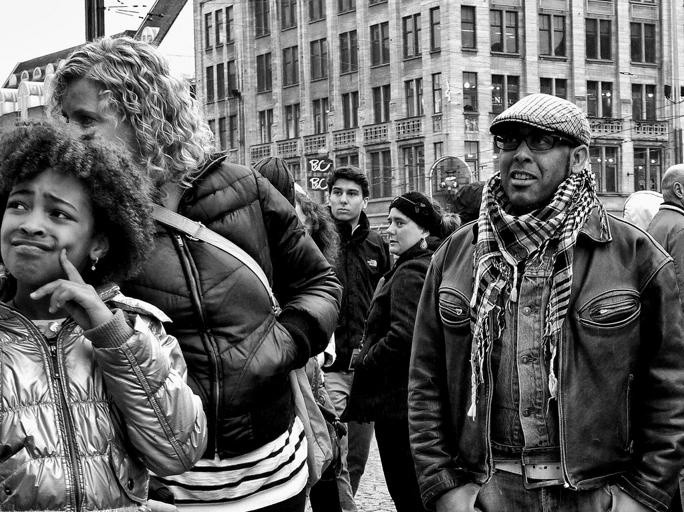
494,130,575,151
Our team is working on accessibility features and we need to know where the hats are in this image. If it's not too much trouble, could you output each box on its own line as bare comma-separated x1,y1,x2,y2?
490,92,591,143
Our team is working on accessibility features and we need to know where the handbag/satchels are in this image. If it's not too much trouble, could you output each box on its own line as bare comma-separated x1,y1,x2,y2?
289,355,346,487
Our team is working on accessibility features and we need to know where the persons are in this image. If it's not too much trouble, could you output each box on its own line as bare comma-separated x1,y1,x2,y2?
450,181,487,226
408,94,684,511
646,163,683,298
0,117,207,510
46,38,343,510
249,156,297,210
623,190,664,231
340,191,461,511
293,189,341,509
327,163,393,500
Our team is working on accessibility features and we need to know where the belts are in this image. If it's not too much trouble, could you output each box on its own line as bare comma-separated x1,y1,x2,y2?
495,457,564,487
